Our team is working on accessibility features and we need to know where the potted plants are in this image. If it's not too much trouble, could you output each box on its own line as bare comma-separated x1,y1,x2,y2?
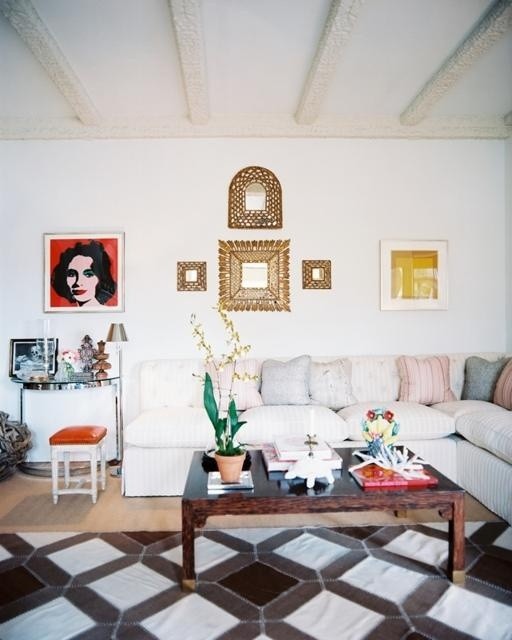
188,299,260,483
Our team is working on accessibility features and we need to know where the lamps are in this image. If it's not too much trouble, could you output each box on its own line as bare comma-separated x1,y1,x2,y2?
107,323,128,476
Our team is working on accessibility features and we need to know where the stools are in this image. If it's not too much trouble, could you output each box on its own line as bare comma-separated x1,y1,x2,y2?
49,425,107,504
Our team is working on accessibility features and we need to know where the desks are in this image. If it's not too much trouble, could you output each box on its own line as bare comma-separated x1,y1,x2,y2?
9,373,120,478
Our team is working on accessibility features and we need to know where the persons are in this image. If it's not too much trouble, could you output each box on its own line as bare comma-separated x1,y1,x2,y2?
52,241,116,307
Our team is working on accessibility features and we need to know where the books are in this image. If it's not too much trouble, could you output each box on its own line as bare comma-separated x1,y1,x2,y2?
262,438,343,475
206,472,253,490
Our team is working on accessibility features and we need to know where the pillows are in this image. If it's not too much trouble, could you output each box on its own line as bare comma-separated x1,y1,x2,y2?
308,358,359,410
191,358,264,410
493,358,511,410
123,405,242,448
461,356,511,403
232,405,352,448
336,401,456,441
456,409,512,465
396,355,457,404
261,354,311,405
428,400,509,419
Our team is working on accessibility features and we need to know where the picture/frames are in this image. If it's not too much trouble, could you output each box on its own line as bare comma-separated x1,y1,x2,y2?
228,166,282,228
218,238,291,312
9,338,58,378
177,261,207,291
44,232,125,313
380,240,448,311
302,260,331,289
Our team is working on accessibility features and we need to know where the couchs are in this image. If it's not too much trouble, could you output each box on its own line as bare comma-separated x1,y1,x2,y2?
122,352,465,496
457,352,511,526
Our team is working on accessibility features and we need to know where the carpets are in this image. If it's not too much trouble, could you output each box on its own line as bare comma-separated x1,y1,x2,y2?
0,462,503,534
